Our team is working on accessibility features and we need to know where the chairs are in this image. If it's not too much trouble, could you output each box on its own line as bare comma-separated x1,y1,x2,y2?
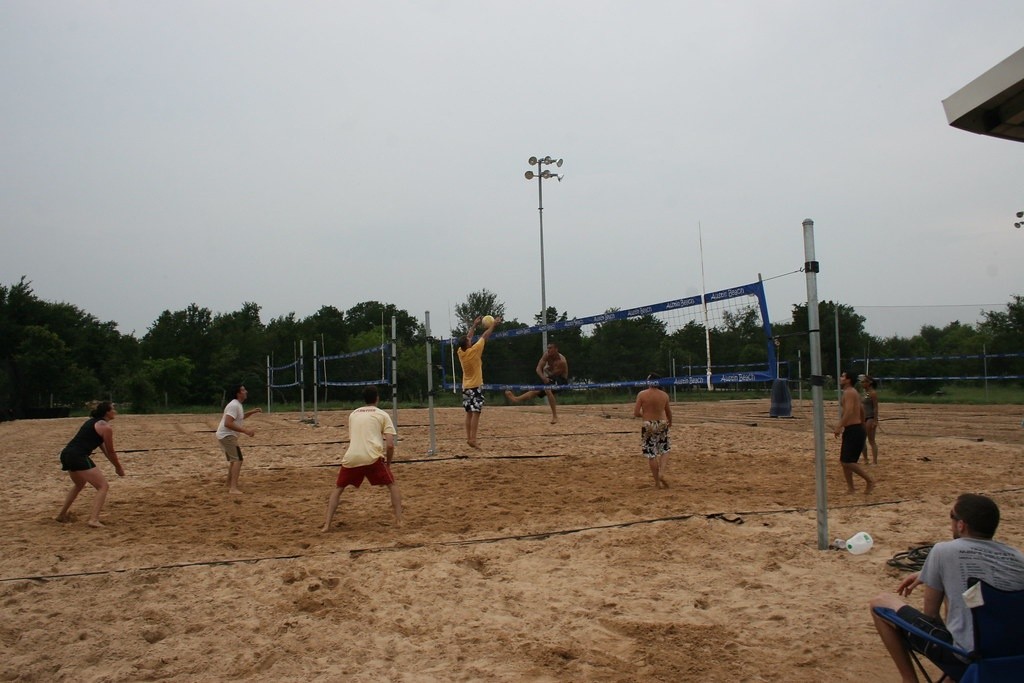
872,576,1024,683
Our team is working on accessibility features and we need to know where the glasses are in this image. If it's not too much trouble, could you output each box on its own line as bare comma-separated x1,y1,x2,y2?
950,510,967,523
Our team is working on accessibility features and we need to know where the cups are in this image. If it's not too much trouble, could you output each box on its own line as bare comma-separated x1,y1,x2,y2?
834,539,846,549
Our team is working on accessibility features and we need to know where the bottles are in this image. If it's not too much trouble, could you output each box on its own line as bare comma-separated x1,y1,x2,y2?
846,532,873,555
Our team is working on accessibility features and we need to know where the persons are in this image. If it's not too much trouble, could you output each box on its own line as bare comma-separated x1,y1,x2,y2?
321,386,402,528
505,341,569,424
217,385,262,494
869,493,1024,683
55,401,125,528
834,371,874,492
861,376,878,464
458,316,501,449
634,373,672,489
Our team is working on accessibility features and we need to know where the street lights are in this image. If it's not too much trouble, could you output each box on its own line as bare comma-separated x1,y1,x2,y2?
525,156,564,404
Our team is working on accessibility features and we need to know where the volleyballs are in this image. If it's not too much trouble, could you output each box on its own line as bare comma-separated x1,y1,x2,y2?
481,315,495,329
